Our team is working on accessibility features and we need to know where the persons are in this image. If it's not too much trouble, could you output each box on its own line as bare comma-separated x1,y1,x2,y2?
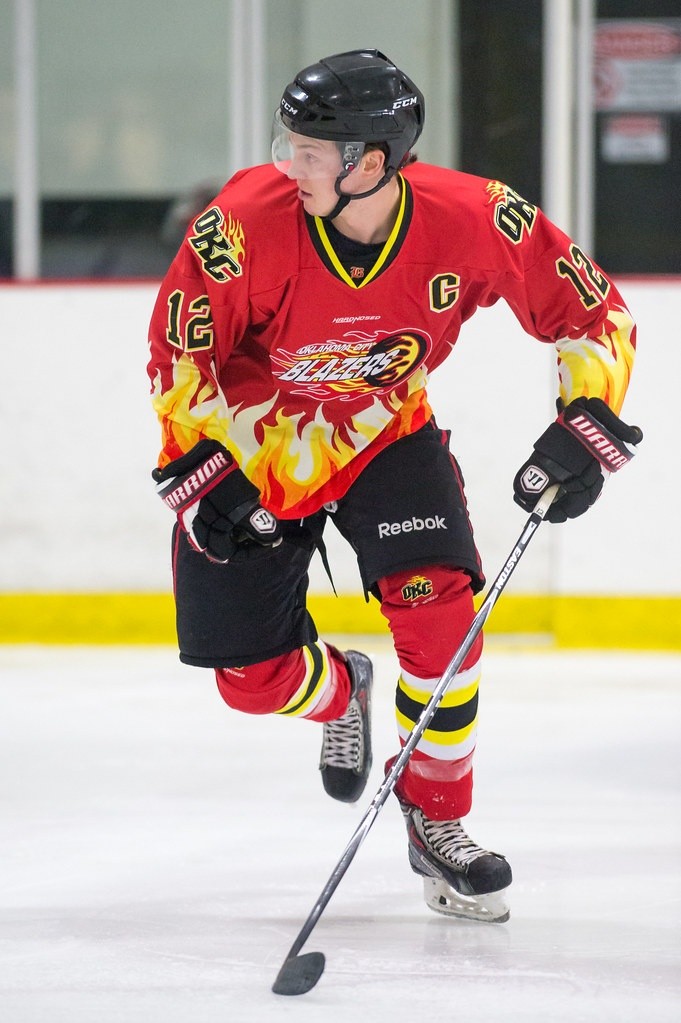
143,47,643,922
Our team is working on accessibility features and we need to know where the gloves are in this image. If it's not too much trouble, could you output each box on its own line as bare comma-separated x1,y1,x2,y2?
151,438,284,564
513,395,643,524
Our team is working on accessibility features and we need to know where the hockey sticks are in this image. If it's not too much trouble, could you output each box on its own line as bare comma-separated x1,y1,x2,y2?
265,476,561,999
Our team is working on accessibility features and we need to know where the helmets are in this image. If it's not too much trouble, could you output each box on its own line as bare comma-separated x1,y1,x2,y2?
279,48,426,144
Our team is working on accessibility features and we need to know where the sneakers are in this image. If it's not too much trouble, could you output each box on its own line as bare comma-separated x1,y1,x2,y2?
384,754,513,923
319,639,373,804
